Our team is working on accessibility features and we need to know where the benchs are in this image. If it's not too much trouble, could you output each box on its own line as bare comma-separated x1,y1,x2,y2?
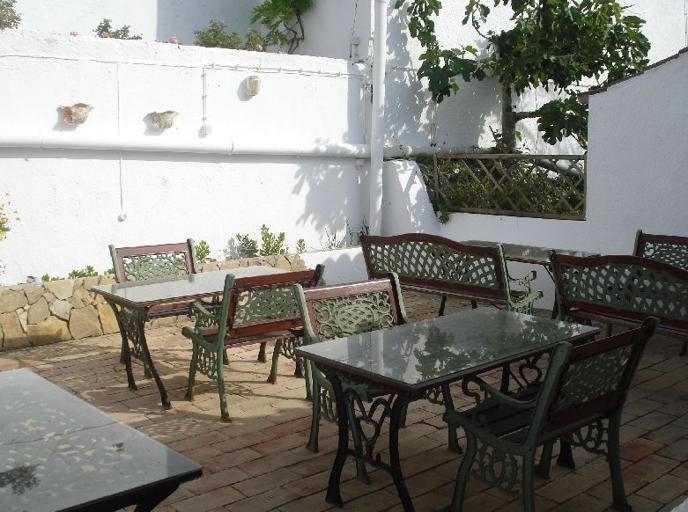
547,249,688,330
605,229,688,311
359,233,544,317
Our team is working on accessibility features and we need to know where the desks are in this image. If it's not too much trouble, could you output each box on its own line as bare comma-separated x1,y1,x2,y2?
0,366,204,511
438,240,600,322
294,304,601,511
90,266,304,410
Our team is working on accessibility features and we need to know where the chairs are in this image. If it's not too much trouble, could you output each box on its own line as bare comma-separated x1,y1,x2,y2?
291,271,464,486
182,263,325,418
108,238,229,379
447,316,661,511
